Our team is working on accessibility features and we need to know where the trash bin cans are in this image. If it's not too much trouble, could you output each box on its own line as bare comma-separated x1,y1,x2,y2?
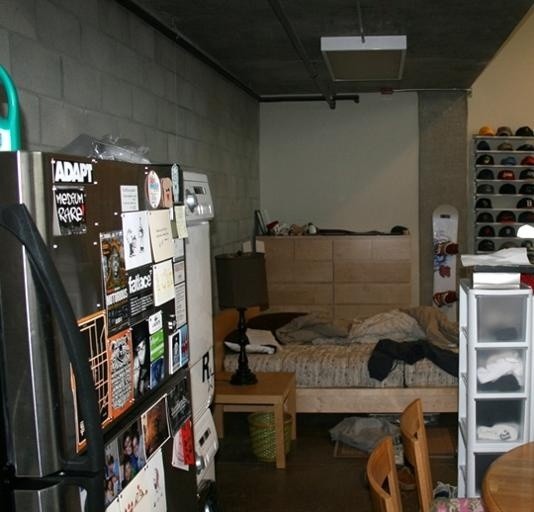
249,413,293,460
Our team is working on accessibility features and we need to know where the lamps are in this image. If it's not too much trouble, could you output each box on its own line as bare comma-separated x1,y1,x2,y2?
215,249,269,385
319,1,407,82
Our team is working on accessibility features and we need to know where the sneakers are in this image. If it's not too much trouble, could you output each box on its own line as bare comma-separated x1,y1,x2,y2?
396,466,417,493
432,481,452,501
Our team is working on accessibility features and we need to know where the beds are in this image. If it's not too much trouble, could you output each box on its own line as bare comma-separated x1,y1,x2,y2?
212,306,458,441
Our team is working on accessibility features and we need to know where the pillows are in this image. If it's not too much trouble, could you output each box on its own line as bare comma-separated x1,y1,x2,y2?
223,327,284,355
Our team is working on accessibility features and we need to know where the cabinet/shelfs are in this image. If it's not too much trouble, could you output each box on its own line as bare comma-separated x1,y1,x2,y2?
456,278,533,499
472,135,534,273
254,235,413,329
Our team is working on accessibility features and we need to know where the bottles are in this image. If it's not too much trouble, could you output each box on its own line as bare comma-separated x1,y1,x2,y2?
308,223,317,235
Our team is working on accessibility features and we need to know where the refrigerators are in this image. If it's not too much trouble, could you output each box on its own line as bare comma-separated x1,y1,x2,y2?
0,151,198,512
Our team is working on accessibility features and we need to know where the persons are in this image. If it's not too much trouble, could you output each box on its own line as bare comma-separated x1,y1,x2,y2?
122,431,139,490
105,456,117,504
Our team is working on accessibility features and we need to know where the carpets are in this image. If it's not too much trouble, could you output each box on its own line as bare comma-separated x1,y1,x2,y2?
334,427,455,460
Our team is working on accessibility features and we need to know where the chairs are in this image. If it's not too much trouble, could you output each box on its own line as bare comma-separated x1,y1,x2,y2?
365,398,486,512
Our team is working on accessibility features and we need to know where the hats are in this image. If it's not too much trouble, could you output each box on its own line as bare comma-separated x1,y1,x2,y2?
475,124,533,263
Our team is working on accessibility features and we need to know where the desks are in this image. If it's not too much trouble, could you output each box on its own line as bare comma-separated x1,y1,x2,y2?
482,441,533,512
213,371,297,469
